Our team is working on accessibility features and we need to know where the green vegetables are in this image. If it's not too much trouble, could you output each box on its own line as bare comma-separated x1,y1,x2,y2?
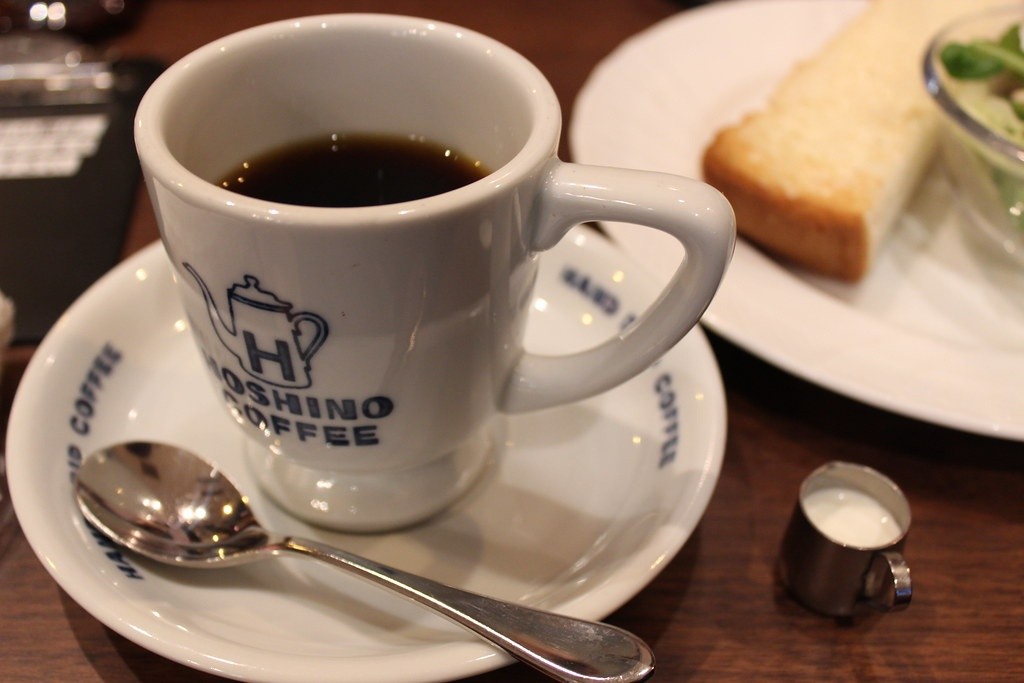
938,20,1024,117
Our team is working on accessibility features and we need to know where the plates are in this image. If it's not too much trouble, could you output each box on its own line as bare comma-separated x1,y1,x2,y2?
6,223,730,683
567,0,1024,441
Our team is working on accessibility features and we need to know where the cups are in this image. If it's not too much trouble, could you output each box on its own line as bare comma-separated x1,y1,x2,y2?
786,460,912,623
134,13,737,534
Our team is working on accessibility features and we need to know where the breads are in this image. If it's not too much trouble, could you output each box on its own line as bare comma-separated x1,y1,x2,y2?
701,0,1024,285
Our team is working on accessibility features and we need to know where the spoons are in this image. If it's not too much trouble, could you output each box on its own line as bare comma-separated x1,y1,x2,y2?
77,441,656,683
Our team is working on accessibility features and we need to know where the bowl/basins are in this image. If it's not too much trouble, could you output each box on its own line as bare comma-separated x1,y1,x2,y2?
923,6,1024,266
